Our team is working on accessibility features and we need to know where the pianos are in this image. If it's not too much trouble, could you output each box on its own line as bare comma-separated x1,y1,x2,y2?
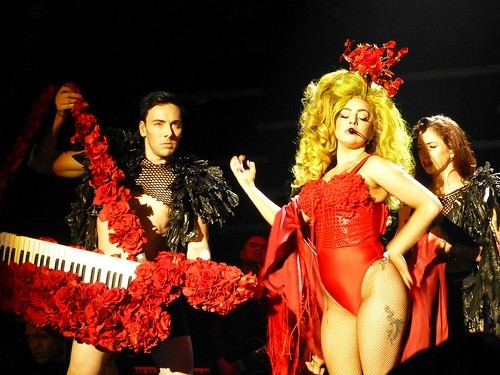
0,231,143,290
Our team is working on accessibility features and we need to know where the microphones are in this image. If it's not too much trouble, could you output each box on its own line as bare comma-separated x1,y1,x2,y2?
349,128,373,150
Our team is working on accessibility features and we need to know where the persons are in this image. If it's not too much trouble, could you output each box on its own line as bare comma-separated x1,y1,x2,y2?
25,322,71,375
230,68,443,375
27,80,214,375
395,113,500,344
212,233,269,375
